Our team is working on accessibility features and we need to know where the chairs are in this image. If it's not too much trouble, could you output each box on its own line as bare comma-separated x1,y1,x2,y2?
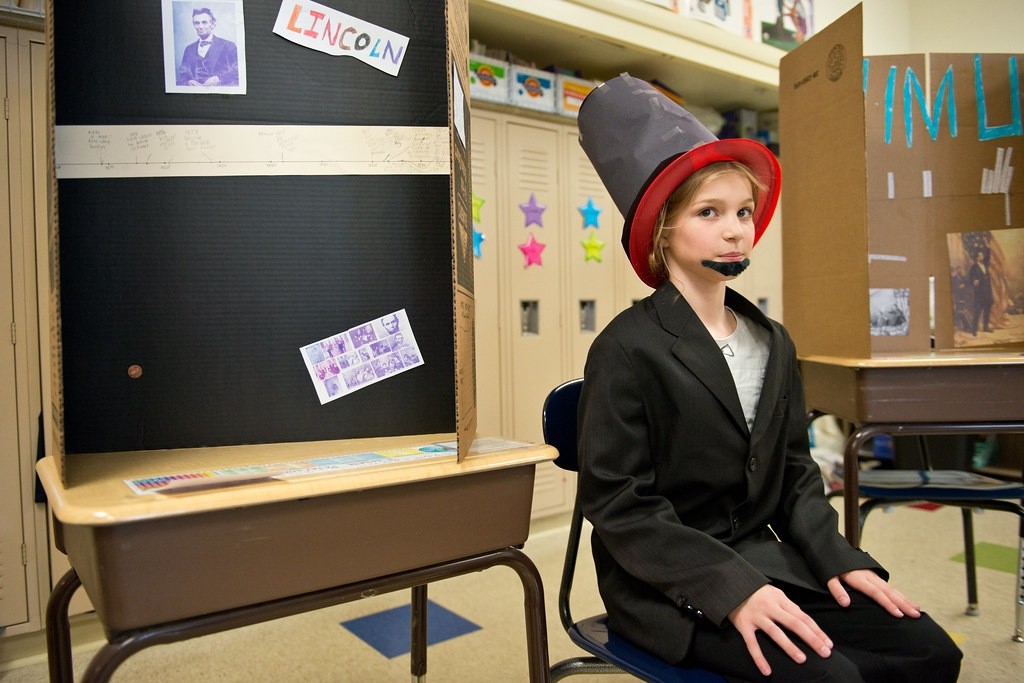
825,410,1024,643
542,378,664,683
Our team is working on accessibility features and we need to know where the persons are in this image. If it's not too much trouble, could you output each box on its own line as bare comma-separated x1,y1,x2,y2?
951,264,967,330
310,314,421,398
176,7,239,86
572,148,965,683
968,250,995,337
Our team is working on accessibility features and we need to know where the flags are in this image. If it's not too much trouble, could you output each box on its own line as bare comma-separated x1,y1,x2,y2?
954,232,1014,333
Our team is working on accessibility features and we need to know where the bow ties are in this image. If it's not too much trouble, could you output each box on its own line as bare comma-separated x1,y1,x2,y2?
200,41,211,46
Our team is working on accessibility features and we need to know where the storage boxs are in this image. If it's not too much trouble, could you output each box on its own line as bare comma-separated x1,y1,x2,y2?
44,0,477,487
467,52,596,120
780,0,1024,358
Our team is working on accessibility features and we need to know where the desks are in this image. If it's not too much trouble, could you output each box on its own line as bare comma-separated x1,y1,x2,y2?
798,352,1024,550
34,436,560,683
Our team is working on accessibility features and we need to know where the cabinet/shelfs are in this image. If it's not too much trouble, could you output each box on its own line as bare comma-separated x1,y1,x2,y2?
469,107,784,525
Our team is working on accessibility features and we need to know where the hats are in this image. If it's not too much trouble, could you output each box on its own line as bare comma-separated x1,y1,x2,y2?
577,76,782,289
